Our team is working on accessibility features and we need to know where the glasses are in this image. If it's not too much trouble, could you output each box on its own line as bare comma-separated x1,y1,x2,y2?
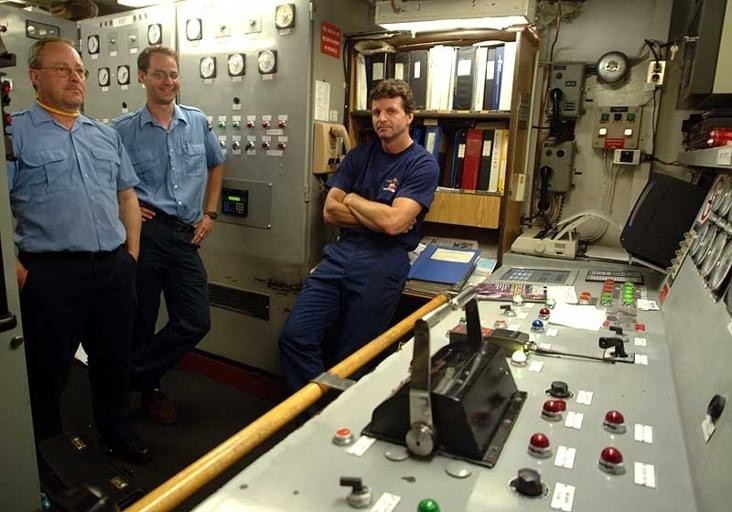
148,70,182,81
37,63,85,76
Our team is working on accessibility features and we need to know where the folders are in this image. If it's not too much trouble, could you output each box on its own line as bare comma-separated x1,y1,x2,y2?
411,50,427,110
368,53,384,109
391,50,409,85
484,49,494,111
405,244,481,298
478,130,494,190
424,125,446,186
455,131,467,188
455,48,474,111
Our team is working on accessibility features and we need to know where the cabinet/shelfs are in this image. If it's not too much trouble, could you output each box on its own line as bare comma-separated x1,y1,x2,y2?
344,22,540,247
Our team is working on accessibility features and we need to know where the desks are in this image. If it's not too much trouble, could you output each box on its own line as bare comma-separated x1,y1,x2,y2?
306,231,502,301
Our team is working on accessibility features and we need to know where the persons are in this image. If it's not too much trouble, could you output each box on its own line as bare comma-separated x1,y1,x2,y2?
279,80,441,395
112,46,223,424
3,38,145,510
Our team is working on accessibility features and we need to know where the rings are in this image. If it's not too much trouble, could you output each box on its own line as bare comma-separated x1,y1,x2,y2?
200,233,209,239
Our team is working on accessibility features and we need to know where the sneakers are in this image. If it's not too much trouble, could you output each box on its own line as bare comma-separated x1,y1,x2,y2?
141,390,177,423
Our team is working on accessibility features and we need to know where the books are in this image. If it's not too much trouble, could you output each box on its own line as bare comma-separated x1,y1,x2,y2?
415,117,508,192
356,39,518,110
407,233,497,298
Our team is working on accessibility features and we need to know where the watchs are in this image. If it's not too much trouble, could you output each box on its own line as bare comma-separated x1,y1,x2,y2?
203,210,219,220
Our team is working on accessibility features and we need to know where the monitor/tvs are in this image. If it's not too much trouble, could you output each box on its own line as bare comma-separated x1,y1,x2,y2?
619,172,706,271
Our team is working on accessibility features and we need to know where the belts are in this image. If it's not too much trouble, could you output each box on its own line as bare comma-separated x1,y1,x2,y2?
176,218,195,233
